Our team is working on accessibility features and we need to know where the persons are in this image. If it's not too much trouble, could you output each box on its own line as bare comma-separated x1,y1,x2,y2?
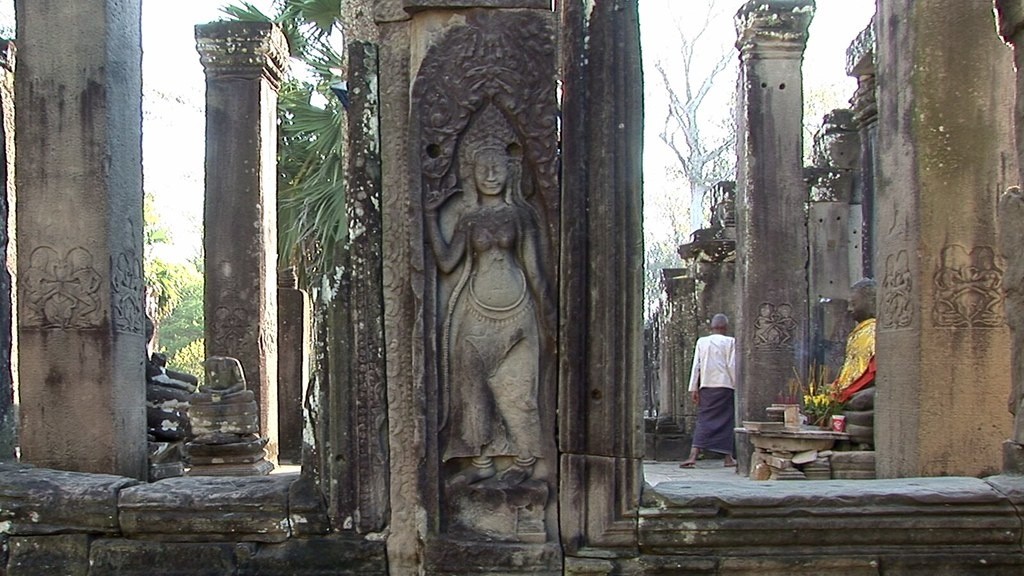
677,313,736,469
426,147,547,483
821,278,876,410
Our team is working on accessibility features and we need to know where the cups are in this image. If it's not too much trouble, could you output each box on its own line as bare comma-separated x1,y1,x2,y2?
644,410,648,418
652,410,656,418
832,414,845,433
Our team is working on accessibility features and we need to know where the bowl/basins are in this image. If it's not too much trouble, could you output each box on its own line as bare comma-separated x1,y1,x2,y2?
766,407,785,423
